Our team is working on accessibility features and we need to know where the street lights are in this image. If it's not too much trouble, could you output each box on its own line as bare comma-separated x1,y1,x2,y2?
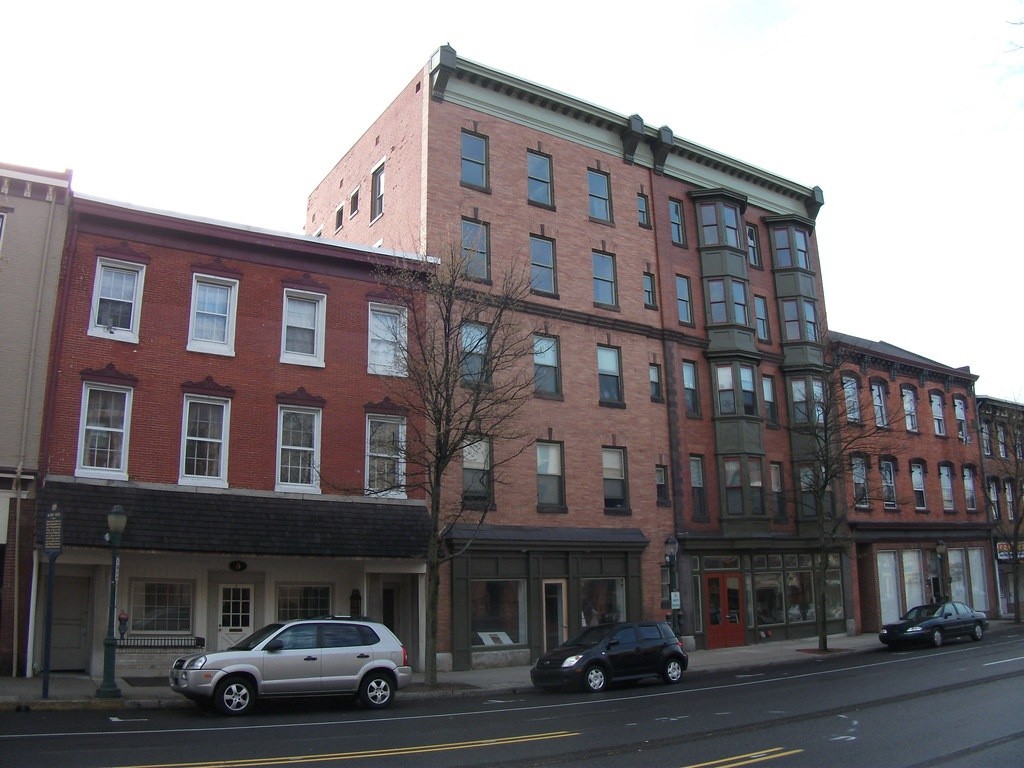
96,505,127,700
935,540,948,602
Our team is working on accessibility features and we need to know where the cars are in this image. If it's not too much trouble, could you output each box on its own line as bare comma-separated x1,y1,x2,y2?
879,602,989,650
529,620,689,694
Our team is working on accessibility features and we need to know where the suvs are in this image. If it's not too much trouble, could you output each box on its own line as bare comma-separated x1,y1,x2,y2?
168,614,412,717
